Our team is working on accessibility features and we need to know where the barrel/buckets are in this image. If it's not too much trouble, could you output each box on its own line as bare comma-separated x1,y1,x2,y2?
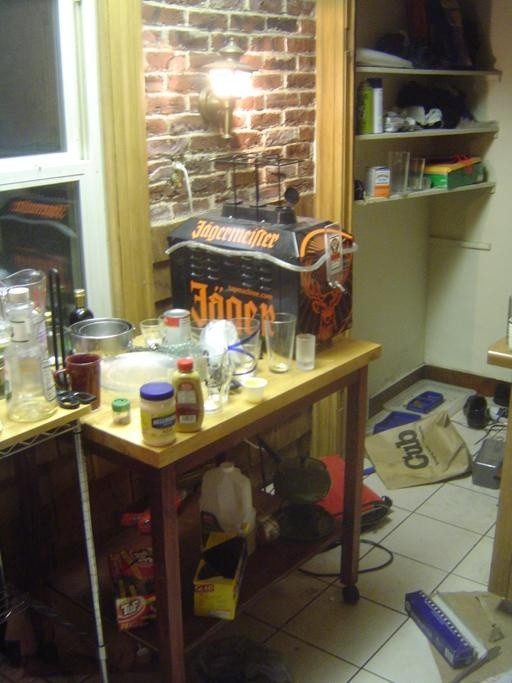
198,462,258,558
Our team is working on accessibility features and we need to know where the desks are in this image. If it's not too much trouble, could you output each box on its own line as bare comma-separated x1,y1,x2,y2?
0,389,114,681
49,321,383,678
483,332,512,610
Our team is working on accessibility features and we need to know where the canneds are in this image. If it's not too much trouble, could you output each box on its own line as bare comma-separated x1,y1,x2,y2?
164,308,192,344
141,382,176,447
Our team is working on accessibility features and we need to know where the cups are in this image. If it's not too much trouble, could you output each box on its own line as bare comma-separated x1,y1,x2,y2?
222,314,261,375
262,310,298,372
387,150,425,192
51,352,101,411
242,377,269,403
294,333,317,371
137,309,217,346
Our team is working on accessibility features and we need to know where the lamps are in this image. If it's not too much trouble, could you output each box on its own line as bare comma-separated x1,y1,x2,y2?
196,34,260,138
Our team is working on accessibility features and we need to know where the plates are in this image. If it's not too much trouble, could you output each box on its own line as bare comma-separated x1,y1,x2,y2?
95,350,176,394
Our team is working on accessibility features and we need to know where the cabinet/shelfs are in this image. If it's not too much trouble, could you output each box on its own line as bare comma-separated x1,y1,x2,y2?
350,46,503,203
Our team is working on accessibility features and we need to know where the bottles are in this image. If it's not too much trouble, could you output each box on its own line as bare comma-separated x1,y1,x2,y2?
357,77,385,134
172,359,206,434
110,399,130,424
66,286,95,325
4,285,58,423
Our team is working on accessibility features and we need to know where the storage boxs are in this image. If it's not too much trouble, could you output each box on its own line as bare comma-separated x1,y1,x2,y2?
105,548,161,633
191,528,249,620
411,151,485,191
